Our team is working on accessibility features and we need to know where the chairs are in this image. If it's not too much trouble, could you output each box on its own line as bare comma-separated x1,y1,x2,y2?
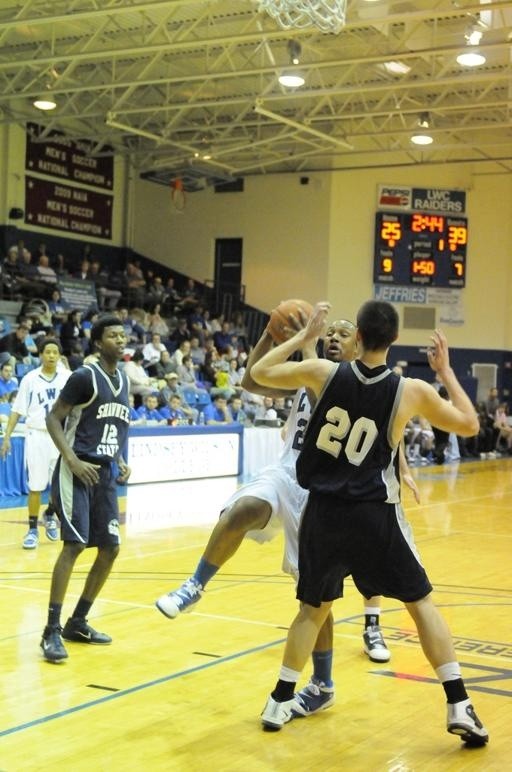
0,254,297,434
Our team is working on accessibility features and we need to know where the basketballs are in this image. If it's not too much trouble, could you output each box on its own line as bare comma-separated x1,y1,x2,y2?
269,299,314,347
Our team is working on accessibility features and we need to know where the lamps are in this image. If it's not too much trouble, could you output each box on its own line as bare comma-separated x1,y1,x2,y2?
411,112,433,145
277,40,306,86
456,30,486,66
33,80,56,110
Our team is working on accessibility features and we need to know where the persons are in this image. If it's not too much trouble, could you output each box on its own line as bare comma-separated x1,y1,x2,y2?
248,298,488,745
3,339,76,550
152,302,359,722
40,316,132,665
394,366,512,465
360,433,420,662
0,239,286,429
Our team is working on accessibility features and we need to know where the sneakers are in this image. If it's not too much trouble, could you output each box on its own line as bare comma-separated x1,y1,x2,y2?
23,529,39,549
363,625,390,663
40,625,68,662
62,616,113,645
156,576,205,619
259,676,336,729
446,696,488,745
43,510,58,541
